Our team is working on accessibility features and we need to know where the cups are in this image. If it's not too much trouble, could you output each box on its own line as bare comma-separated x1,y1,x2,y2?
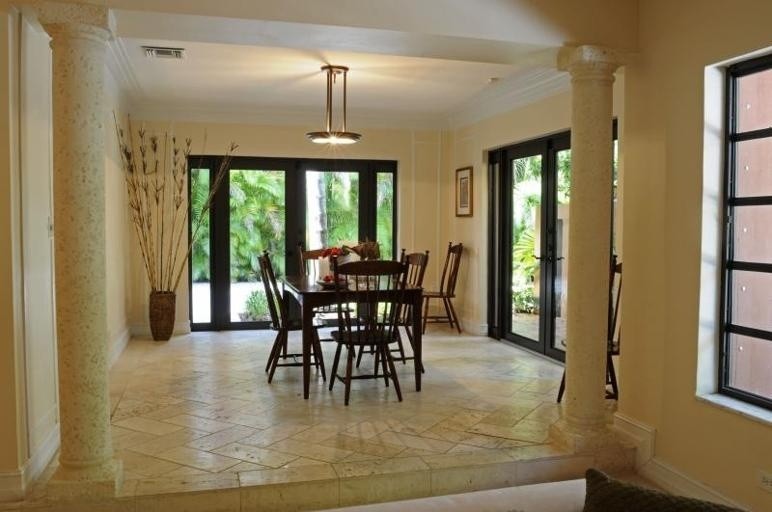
318,256,330,279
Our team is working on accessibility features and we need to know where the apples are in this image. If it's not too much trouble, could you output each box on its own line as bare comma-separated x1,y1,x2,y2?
323,274,345,281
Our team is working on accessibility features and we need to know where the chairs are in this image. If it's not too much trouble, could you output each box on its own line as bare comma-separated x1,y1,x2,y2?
257,242,463,406
556,253,623,404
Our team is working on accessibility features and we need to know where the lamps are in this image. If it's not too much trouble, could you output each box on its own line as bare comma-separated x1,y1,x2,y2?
306,66,361,145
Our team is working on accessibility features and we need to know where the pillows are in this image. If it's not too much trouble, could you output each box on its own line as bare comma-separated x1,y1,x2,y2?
584,467,747,512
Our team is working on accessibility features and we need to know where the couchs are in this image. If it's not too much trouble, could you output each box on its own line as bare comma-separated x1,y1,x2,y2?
322,477,587,512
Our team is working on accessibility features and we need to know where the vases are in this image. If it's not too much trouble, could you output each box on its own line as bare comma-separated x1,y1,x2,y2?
150,290,176,341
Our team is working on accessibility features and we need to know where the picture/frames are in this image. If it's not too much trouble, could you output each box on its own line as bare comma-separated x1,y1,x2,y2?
455,167,474,216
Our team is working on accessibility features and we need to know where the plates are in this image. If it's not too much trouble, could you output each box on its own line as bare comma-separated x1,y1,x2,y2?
315,277,354,289
